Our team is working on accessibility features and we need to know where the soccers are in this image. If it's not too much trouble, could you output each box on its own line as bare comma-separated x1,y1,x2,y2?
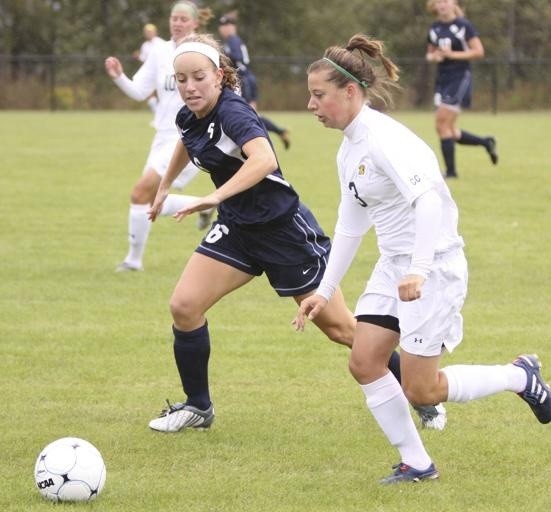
34,438,106,504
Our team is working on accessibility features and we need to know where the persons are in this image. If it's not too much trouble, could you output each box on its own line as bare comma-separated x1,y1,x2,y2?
291,34,551,485
147,32,446,432
425,0,499,179
218,10,291,149
137,24,166,112
103,0,242,275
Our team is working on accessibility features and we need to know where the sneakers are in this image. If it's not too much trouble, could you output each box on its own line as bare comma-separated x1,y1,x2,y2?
148,398,215,433
113,262,142,272
375,462,440,485
280,128,290,150
487,136,499,166
513,353,551,424
198,207,216,231
415,402,449,431
